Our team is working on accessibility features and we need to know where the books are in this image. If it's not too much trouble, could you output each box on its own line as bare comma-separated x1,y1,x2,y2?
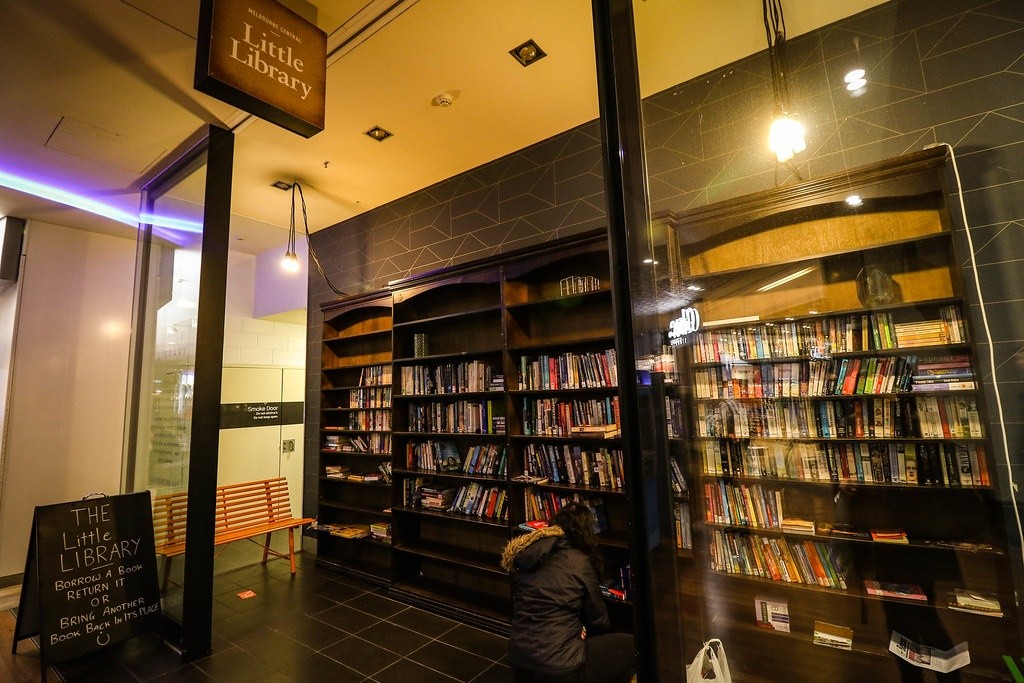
324,409,391,431
520,394,621,439
517,487,608,532
513,345,620,390
403,436,509,477
317,513,392,545
596,556,637,601
349,387,393,408
383,506,392,513
325,460,392,483
400,359,504,395
360,363,393,387
661,294,1006,675
401,475,511,521
407,398,506,433
413,332,428,358
324,432,391,453
523,443,624,489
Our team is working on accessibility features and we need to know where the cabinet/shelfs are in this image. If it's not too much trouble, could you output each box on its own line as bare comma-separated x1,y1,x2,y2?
316,143,1023,683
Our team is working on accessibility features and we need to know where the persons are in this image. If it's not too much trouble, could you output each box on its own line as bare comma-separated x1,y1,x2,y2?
496,500,640,683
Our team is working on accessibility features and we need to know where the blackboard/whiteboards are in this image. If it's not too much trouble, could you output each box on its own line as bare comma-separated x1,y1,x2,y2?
34,490,170,683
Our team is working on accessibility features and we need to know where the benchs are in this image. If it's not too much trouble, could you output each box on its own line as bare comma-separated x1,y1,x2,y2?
149,476,317,595
